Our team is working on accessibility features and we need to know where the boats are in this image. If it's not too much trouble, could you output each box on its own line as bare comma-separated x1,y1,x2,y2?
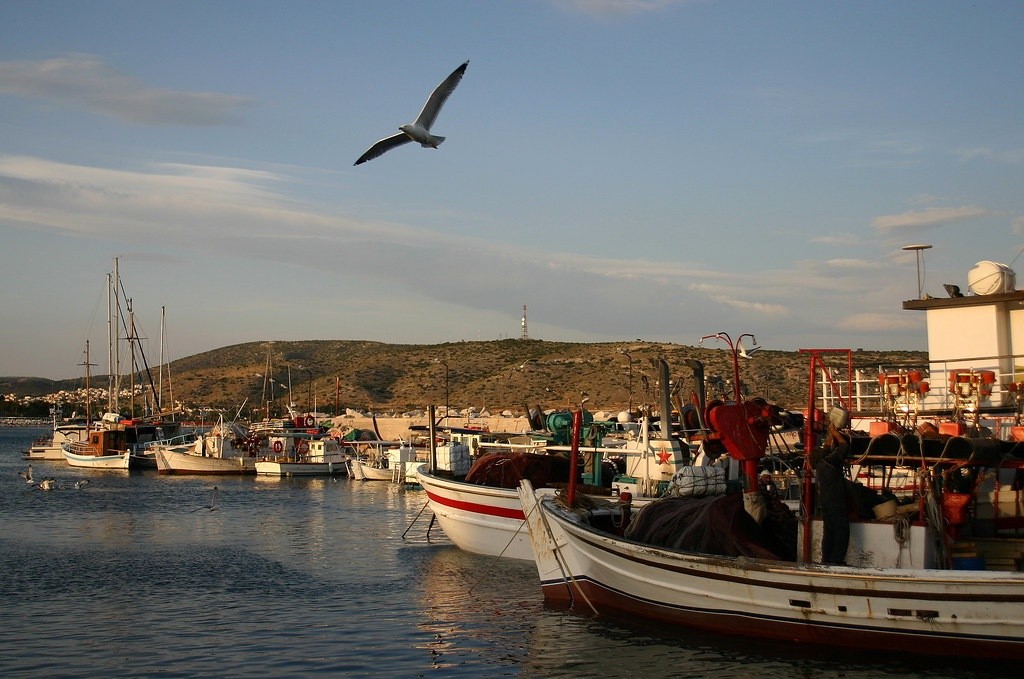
19,243,1024,673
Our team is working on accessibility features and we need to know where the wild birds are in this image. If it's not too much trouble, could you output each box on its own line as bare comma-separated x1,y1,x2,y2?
353,60,470,167
738,341,761,359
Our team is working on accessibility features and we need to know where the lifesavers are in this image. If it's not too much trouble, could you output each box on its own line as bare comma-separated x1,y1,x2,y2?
299,442,309,453
274,441,283,453
